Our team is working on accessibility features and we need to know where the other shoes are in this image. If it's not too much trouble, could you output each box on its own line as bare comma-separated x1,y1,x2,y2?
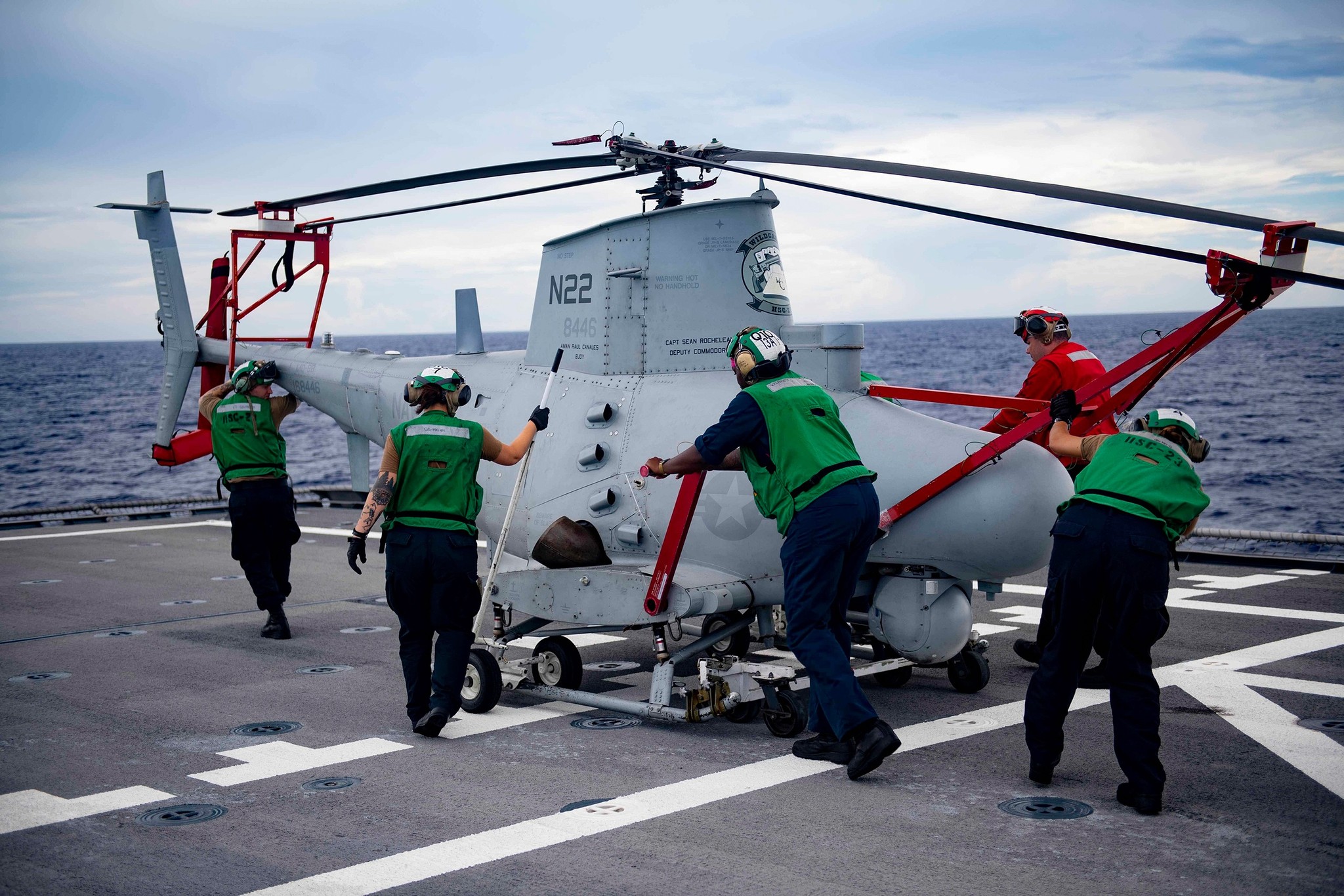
1078,662,1111,689
847,720,903,778
1013,638,1042,663
792,731,849,763
411,708,451,737
1030,760,1056,784
1116,781,1164,816
260,608,294,639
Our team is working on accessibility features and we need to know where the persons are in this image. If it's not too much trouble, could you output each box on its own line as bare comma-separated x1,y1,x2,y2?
347,366,550,734
644,325,899,779
979,303,1124,690
1020,387,1213,816
199,358,306,639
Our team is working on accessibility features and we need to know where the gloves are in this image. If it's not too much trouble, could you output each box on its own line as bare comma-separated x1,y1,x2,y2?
346,537,367,576
528,405,550,431
1049,390,1083,432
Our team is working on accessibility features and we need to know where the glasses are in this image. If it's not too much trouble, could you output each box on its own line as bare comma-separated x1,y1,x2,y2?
255,360,280,379
754,349,791,379
1013,316,1025,334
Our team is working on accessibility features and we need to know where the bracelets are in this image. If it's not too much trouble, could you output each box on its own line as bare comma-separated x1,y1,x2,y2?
659,458,671,475
1054,417,1071,432
351,527,368,540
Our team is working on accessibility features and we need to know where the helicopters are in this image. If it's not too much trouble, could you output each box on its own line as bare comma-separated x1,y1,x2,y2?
93,122,1344,740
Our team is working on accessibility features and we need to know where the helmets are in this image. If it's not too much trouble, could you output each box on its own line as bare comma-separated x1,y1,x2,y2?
1144,408,1203,442
231,360,282,387
726,326,786,361
1018,304,1070,342
412,365,460,392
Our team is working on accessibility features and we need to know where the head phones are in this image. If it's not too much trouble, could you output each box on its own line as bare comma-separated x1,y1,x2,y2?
236,360,266,392
734,326,757,378
1016,313,1050,335
1128,410,1209,462
402,367,473,407
